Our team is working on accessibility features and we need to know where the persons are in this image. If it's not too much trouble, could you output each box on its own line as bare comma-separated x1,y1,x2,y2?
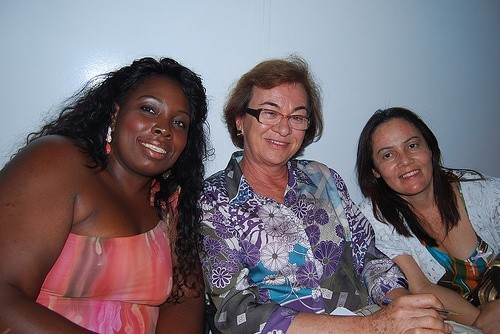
0,58,217,334
200,54,453,334
358,108,500,334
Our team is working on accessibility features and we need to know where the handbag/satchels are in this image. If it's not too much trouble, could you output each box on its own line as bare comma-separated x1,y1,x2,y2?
466,253,500,307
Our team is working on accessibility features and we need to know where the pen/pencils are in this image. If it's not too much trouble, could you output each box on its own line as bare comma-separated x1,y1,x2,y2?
383,298,461,316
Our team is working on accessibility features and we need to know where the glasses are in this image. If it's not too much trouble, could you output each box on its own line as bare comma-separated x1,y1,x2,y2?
246,107,312,131
